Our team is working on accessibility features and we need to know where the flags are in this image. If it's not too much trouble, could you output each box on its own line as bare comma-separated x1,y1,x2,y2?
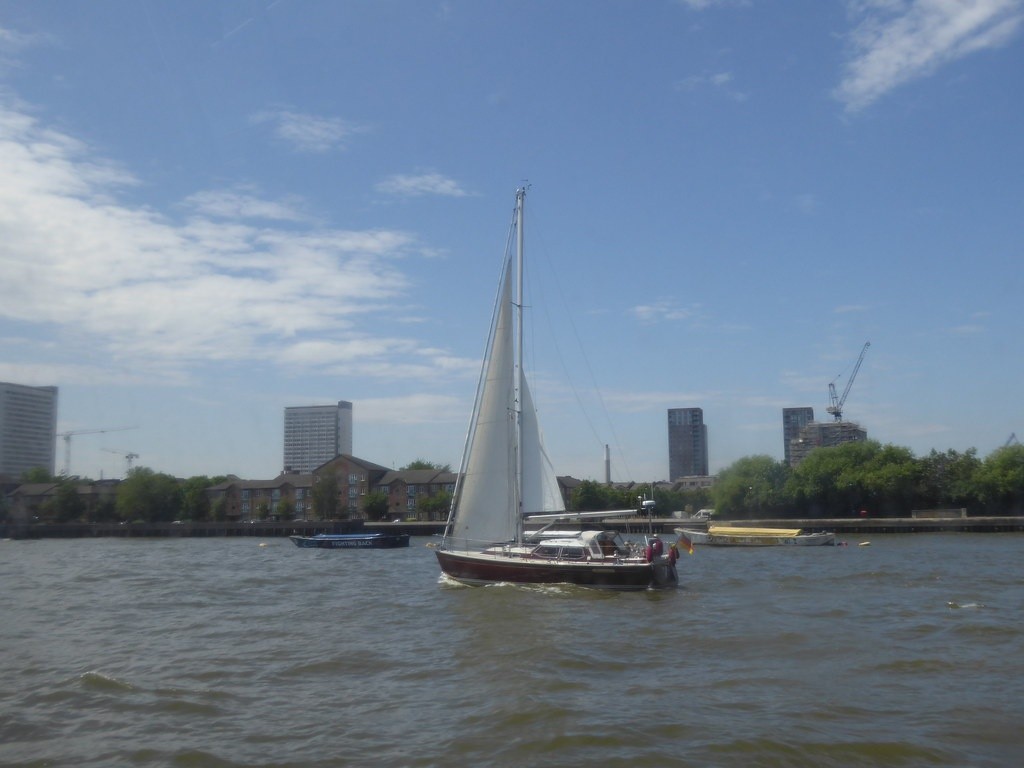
677,532,696,554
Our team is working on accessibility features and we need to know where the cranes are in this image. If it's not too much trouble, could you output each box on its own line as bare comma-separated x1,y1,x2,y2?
825,341,872,420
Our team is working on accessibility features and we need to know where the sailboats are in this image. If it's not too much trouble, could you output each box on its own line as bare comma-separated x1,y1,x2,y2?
426,187,681,589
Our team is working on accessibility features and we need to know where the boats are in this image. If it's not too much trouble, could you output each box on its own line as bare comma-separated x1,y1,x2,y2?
289,534,409,549
674,526,835,546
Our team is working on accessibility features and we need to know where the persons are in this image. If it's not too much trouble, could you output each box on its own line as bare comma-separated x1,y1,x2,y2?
653,533,664,555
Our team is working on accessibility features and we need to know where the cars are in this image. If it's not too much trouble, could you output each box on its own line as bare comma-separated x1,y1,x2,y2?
690,509,715,520
